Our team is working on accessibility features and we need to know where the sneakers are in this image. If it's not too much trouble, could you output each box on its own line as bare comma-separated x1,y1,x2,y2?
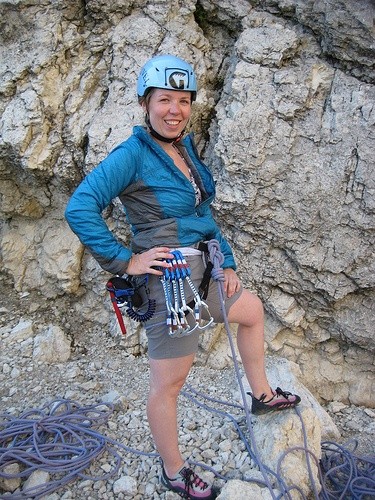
158,458,217,499
246,387,301,415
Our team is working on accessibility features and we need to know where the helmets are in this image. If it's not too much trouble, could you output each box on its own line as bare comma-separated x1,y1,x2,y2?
136,55,198,103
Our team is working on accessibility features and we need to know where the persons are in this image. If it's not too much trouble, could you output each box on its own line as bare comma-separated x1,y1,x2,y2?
66,55,302,500
